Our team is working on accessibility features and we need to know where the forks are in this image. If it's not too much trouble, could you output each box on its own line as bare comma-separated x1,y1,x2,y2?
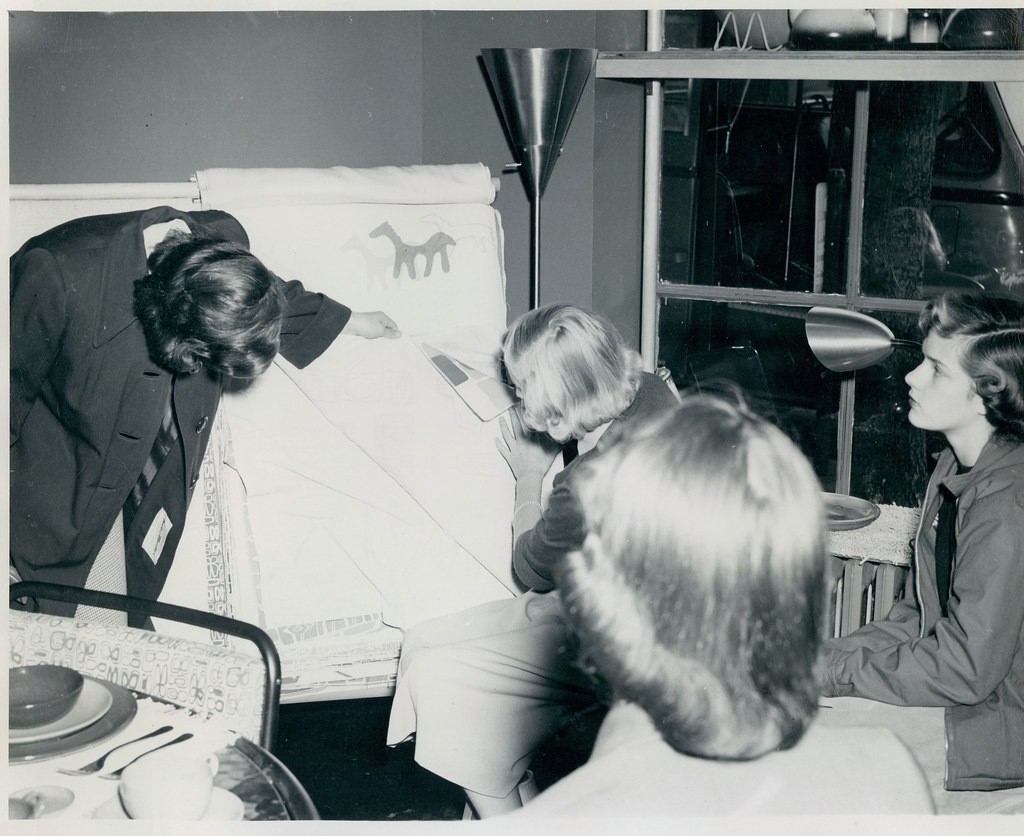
96,732,194,780
56,725,174,776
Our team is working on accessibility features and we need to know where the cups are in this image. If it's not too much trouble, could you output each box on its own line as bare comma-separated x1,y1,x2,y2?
910,13,941,43
8,791,45,819
119,752,220,820
874,9,907,42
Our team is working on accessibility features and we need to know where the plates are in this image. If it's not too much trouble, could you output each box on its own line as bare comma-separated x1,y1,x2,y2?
9,678,113,744
9,674,138,766
821,492,881,531
91,786,245,821
8,786,75,816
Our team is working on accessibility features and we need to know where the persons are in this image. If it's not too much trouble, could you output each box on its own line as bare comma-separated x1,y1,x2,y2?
10,206,402,632
814,289,1024,792
386,300,681,834
479,376,938,835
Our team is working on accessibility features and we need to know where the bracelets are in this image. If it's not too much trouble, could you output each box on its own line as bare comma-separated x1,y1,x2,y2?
510,501,544,527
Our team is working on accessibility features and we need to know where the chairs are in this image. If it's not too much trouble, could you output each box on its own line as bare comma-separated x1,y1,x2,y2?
9,581,281,756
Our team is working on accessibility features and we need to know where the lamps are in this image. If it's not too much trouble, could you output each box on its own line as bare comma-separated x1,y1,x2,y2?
479,44,601,311
805,307,922,371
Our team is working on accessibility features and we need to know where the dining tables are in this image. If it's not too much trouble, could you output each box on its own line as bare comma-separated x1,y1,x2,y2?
10,664,321,821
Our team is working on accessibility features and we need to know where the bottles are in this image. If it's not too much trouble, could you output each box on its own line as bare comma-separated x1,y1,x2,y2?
791,9,878,50
940,9,1024,50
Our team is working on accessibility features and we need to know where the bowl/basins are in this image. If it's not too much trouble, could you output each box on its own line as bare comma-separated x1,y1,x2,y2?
712,10,791,49
9,665,84,729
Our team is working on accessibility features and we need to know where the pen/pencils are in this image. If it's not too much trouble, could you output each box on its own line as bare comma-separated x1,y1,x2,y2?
188,472,199,491
